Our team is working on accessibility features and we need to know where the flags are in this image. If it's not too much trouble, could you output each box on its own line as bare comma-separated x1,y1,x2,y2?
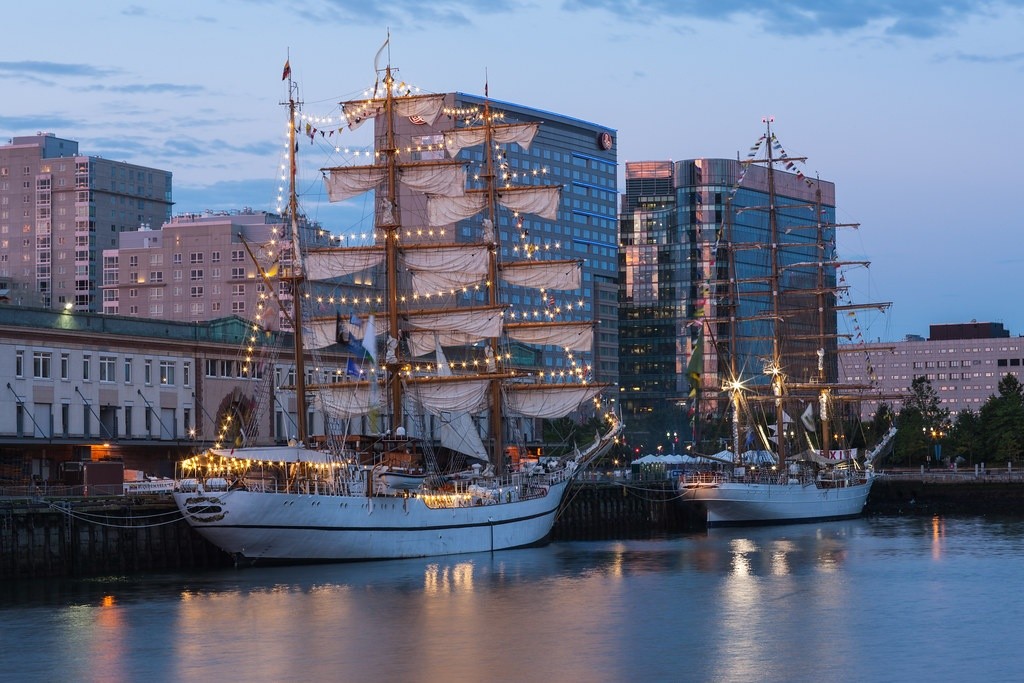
359,314,381,365
281,61,290,81
346,331,361,353
348,311,364,328
345,357,368,379
332,309,354,344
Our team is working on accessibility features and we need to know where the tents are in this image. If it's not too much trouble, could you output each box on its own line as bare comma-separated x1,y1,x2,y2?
632,449,734,469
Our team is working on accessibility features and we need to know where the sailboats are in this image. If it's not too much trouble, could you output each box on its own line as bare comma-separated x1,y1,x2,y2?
676,112,918,524
177,26,627,558
